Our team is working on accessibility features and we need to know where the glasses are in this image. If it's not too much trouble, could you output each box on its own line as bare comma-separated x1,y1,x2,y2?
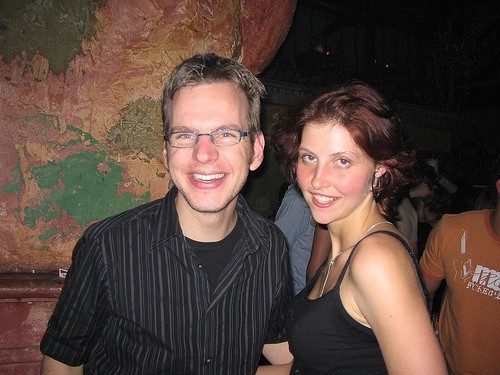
163,129,252,148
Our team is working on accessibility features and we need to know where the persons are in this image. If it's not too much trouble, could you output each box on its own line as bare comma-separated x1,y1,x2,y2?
255,83,450,375
40,53,294,375
419,156,500,375
268,104,447,315
